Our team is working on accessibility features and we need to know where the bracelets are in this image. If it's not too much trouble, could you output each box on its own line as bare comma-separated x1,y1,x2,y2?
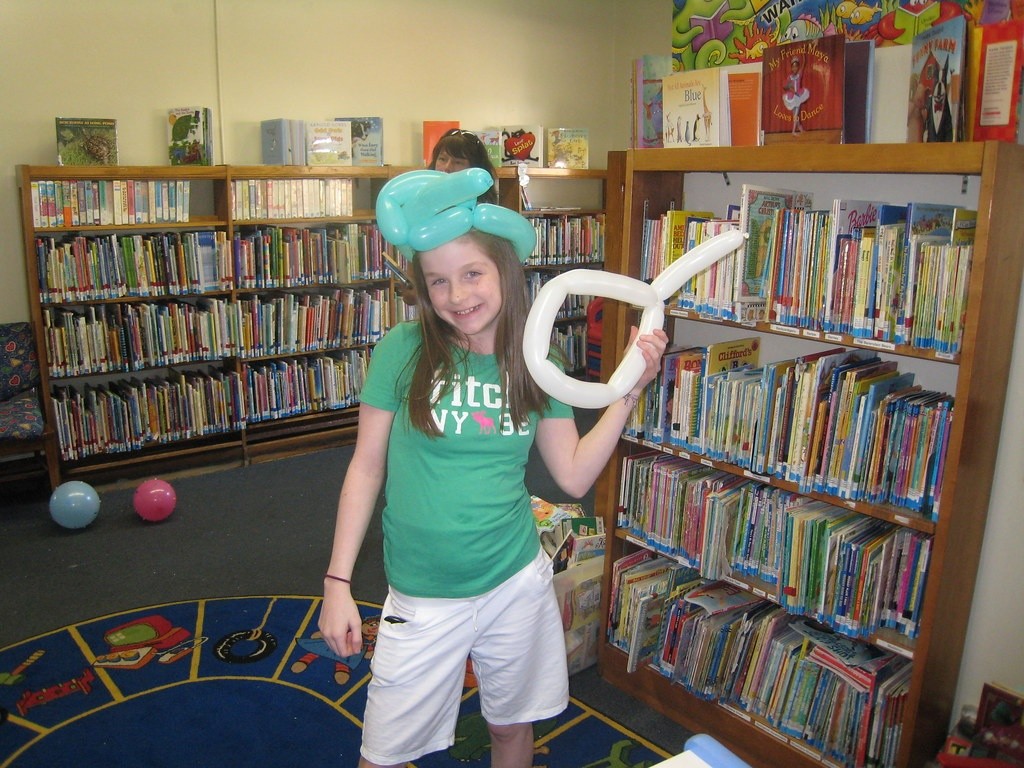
325,571,352,587
623,393,639,408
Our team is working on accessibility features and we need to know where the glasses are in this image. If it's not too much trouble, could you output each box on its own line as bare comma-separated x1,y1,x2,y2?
447,129,481,145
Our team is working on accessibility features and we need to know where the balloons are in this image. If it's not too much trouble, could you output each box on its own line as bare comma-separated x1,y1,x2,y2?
376,167,537,262
48,480,102,529
523,229,750,409
134,478,177,521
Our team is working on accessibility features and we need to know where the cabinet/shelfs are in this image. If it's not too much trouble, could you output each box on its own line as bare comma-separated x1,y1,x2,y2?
491,167,607,376
595,138,1024,768
15,155,426,496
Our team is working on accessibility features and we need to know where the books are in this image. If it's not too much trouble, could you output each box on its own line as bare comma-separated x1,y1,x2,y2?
260,118,384,165
976,681,1024,760
55,117,118,166
629,14,1024,150
548,128,589,169
382,251,417,290
523,215,606,373
29,178,420,463
423,121,543,169
607,184,978,768
166,107,214,166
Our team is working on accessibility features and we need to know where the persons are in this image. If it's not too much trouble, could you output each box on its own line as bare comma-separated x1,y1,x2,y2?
382,128,498,307
317,207,668,768
782,46,810,137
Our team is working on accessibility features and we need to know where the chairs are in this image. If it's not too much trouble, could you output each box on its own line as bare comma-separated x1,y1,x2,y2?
0,322,60,494
